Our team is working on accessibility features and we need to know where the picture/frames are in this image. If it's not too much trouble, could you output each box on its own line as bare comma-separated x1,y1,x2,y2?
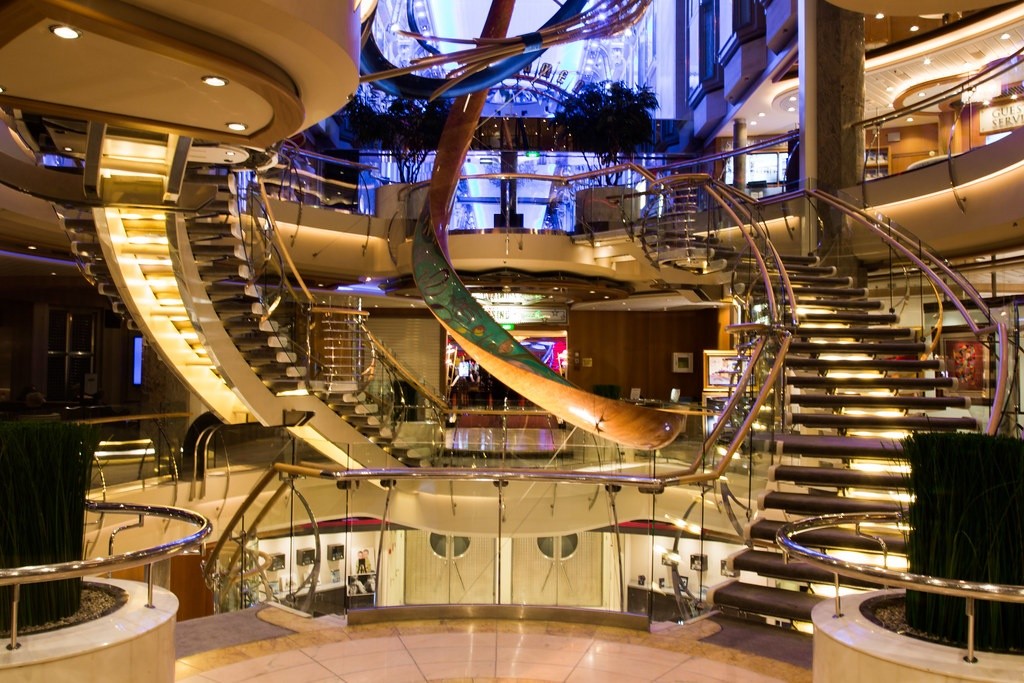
931,322,997,407
704,350,759,393
678,576,688,590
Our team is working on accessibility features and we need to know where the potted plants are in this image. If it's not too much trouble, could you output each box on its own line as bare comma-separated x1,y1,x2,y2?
812,428,1023,683
544,78,664,231
346,90,454,221
0,418,178,683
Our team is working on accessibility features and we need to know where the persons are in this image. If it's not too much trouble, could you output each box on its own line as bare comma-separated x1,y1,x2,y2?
356,549,371,574
470,359,480,382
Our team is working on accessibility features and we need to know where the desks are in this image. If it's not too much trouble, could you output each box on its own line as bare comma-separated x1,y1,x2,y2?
652,408,713,478
446,407,557,468
